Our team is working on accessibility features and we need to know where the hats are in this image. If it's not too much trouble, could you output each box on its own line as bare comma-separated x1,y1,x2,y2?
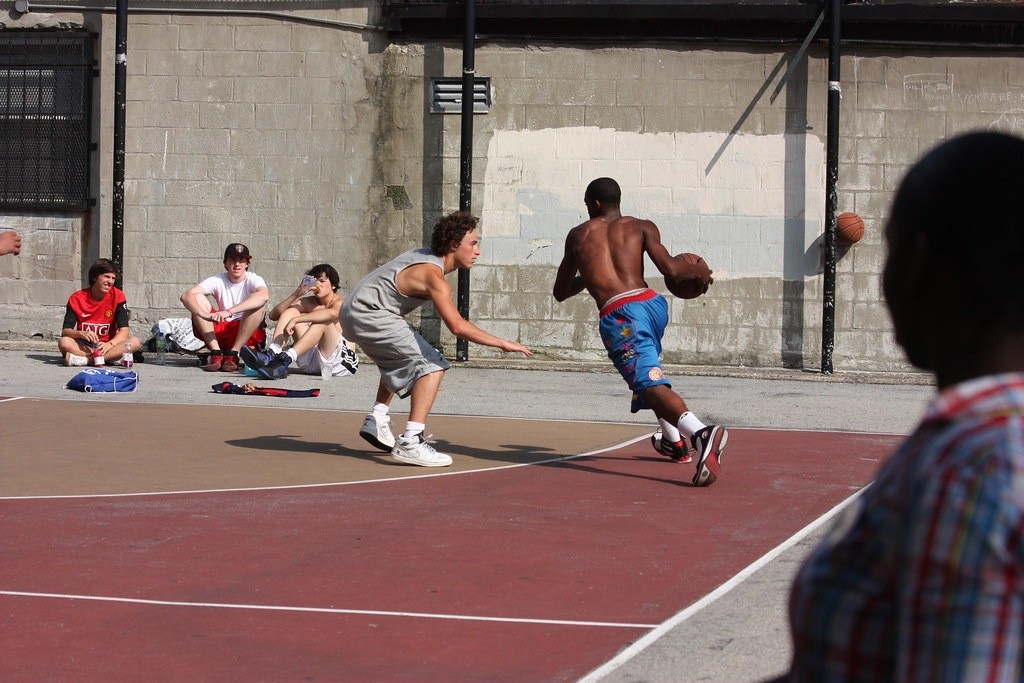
224,243,252,260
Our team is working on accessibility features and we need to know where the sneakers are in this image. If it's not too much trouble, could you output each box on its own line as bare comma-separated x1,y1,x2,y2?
205,350,239,372
691,425,728,486
65,352,88,366
359,414,396,450
241,345,277,371
391,434,453,467
258,352,292,379
652,431,692,463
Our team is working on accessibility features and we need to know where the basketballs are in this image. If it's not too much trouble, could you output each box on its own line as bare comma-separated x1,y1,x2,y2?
838,212,865,245
663,254,711,298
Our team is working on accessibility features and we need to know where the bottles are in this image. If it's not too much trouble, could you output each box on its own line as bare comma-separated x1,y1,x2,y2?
92,340,105,368
303,275,320,293
156,333,166,365
122,343,133,369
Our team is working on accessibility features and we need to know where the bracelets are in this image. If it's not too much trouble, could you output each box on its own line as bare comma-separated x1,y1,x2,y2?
109,341,113,347
226,309,233,318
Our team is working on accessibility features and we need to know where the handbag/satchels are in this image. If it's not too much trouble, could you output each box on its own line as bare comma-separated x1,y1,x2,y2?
67,368,141,394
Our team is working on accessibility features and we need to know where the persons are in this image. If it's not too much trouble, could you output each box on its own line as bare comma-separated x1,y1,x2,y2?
764,130,1024,683
0,230,22,256
338,211,533,467
180,242,268,371
58,258,140,366
553,177,728,485
240,264,358,380
